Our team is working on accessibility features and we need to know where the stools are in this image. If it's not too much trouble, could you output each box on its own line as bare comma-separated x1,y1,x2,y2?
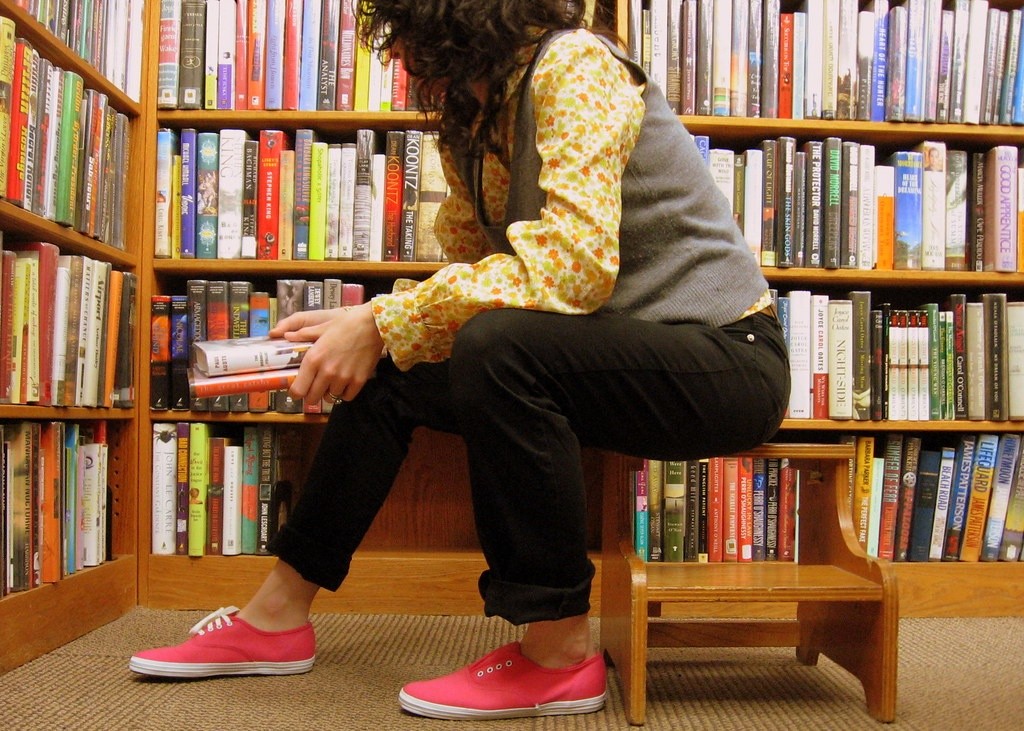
599,440,899,722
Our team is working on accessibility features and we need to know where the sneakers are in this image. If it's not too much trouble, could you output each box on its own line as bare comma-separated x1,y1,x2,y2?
397,641,607,721
129,606,316,677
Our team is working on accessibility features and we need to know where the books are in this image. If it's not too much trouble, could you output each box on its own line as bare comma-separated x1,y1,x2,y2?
0,0,1023,600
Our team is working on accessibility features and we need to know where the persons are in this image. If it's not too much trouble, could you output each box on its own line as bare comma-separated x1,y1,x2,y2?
127,0,791,720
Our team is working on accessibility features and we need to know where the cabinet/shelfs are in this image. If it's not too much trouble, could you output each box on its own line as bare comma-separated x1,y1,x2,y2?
137,0,1024,618
0,0,151,676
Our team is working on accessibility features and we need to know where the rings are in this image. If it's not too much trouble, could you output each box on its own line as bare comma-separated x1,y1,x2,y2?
328,390,343,405
